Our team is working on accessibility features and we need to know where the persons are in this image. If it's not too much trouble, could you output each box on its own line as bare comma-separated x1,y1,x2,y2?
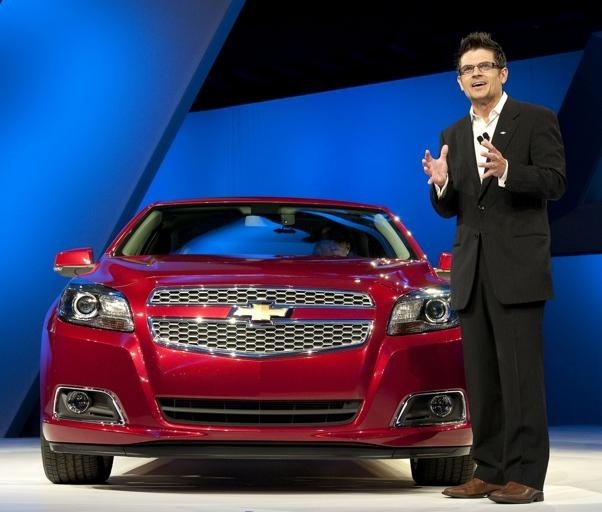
421,33,567,503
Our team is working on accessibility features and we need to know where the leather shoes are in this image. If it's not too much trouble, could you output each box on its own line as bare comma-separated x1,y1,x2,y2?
442,477,544,504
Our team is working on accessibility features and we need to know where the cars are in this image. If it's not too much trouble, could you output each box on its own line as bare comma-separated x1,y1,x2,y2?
40,196,474,484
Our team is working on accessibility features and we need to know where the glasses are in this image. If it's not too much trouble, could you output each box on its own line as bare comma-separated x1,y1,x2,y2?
459,62,502,76
